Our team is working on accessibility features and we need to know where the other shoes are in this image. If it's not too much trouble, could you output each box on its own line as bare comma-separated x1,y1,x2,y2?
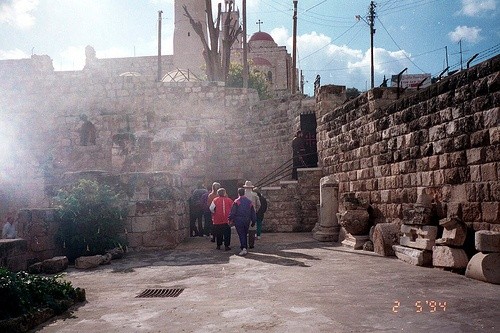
257,235,261,240
225,246,231,250
216,245,220,250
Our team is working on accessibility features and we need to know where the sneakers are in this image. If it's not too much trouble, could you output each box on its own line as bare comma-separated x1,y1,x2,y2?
238,248,247,255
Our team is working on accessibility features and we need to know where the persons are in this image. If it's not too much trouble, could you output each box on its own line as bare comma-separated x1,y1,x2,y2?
209,187,235,251
251,187,267,239
188,186,211,238
291,130,309,179
206,183,226,243
241,181,261,249
203,185,217,238
2,216,16,239
229,187,256,256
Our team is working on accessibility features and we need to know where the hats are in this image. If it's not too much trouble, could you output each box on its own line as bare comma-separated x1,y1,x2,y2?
243,180,254,188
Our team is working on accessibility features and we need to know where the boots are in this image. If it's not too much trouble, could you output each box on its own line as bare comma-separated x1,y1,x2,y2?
211,237,215,242
